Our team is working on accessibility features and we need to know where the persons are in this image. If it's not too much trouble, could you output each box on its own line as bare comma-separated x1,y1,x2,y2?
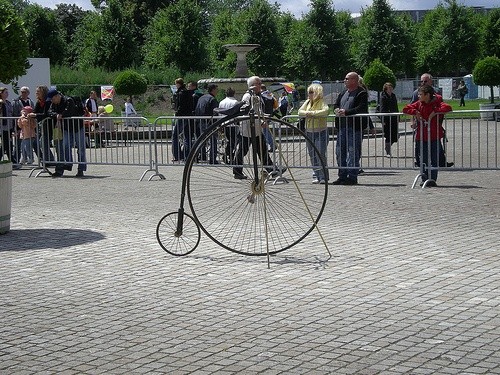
124,97,137,130
0,86,98,178
171,74,467,185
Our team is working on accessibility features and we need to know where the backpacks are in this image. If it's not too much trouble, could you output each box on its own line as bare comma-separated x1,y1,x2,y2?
281,97,288,108
64,95,86,116
259,94,274,114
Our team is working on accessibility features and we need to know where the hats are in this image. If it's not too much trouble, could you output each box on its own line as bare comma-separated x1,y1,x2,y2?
47,88,58,98
22,105,33,113
20,86,31,95
207,83,218,92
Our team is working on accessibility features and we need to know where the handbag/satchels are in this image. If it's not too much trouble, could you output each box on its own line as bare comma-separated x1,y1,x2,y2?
297,118,305,132
52,124,63,141
461,88,468,94
375,105,380,118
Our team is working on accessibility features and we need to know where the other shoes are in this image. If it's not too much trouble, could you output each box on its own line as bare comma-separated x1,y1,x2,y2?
27,158,35,164
419,179,437,187
12,164,22,169
340,176,357,185
444,162,454,167
272,168,287,178
357,169,364,176
209,160,219,164
319,179,325,183
234,173,247,179
268,142,279,152
76,171,83,176
332,175,348,185
52,172,63,177
19,159,26,164
387,153,393,158
311,180,319,184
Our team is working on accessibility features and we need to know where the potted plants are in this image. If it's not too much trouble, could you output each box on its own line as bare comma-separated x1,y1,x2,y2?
0,5,30,234
472,55,500,121
362,58,397,122
297,85,307,107
114,70,148,126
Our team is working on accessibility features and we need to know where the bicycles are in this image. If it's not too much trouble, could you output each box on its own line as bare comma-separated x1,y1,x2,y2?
155,86,329,257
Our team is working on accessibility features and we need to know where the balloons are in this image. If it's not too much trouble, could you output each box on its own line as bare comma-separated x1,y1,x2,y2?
104,105,113,113
